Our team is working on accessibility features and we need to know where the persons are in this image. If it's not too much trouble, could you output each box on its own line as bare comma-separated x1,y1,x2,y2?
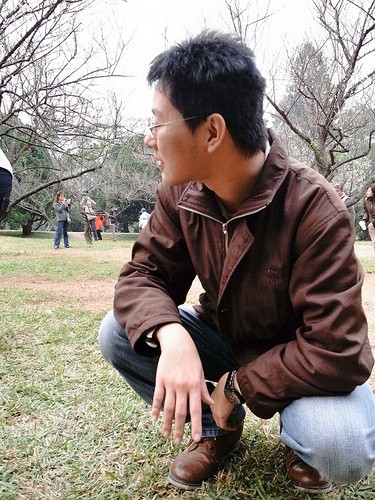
53,191,70,249
98,34,375,492
95,210,104,240
106,204,116,241
138,208,149,231
334,184,355,222
363,183,375,274
79,190,96,245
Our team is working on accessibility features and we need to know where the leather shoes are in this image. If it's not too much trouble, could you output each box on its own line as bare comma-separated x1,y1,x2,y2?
282,443,334,495
167,424,243,491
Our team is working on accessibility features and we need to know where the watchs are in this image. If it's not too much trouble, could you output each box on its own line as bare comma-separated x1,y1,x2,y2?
224,369,244,405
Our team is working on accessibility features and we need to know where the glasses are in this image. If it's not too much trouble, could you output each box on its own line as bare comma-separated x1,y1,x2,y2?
146,116,202,139
